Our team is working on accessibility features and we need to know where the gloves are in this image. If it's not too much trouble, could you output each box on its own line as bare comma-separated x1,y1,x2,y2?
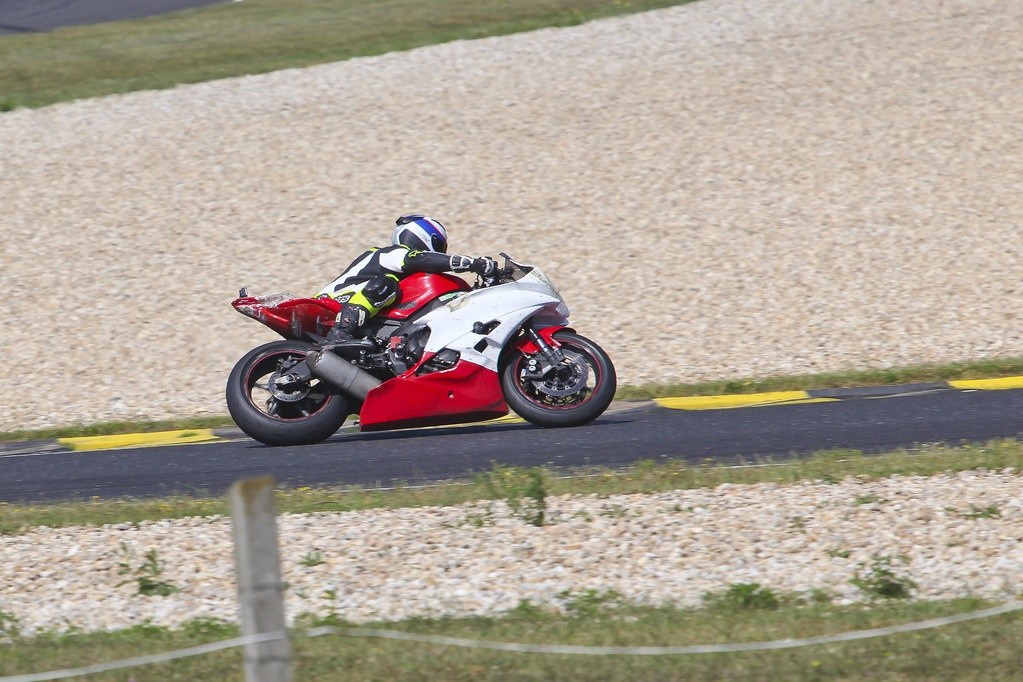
451,255,494,275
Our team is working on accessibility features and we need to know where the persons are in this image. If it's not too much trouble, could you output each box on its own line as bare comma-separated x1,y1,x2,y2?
311,214,498,365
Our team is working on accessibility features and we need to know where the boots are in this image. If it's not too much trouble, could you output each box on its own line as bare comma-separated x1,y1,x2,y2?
319,303,375,357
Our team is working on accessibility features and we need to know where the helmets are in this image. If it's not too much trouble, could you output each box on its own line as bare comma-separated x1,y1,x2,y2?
392,215,447,254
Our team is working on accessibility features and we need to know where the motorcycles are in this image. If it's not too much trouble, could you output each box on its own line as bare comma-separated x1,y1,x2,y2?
225,252,618,446
